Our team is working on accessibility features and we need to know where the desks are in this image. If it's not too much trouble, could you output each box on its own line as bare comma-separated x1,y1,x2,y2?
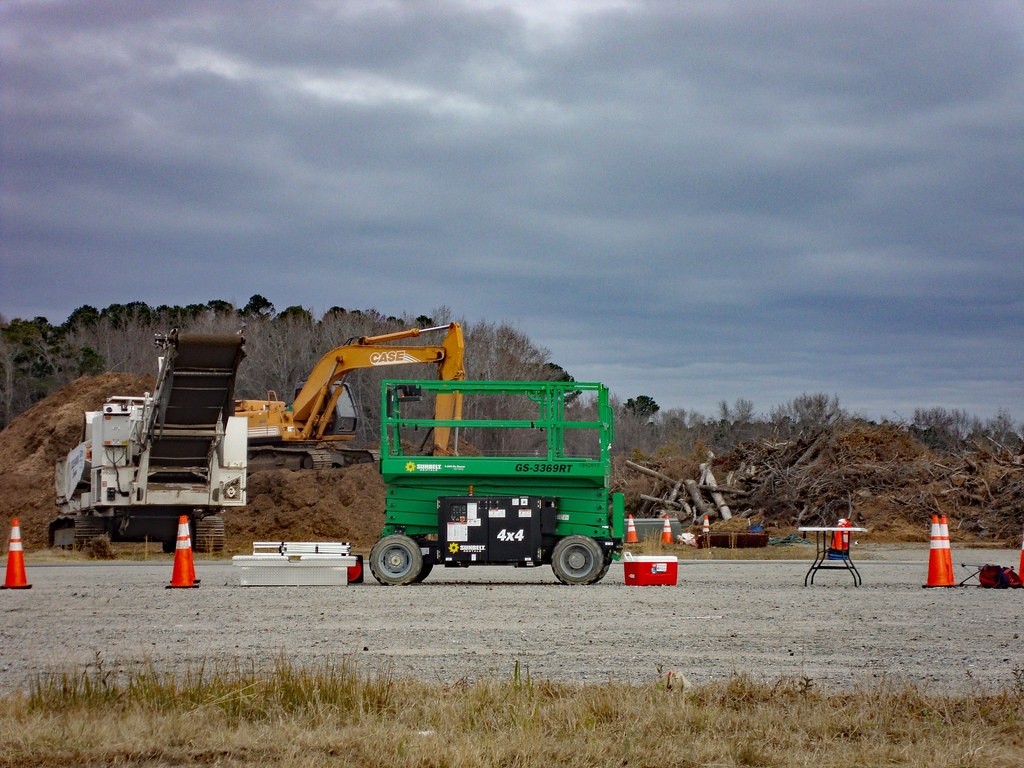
798,526,868,587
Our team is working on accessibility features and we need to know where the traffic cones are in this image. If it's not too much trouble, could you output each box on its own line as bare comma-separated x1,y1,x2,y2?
923,515,957,587
832,522,852,550
627,514,638,543
661,516,673,544
1017,542,1024,588
702,516,710,532
166,516,201,589
0,519,33,589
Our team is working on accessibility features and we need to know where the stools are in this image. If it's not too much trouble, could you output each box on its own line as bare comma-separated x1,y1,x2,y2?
959,563,987,587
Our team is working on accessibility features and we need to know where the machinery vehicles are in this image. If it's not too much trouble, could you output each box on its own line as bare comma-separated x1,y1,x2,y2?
46,327,249,553
235,323,466,472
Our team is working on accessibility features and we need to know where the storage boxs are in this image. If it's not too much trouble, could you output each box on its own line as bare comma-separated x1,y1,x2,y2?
623,551,678,585
